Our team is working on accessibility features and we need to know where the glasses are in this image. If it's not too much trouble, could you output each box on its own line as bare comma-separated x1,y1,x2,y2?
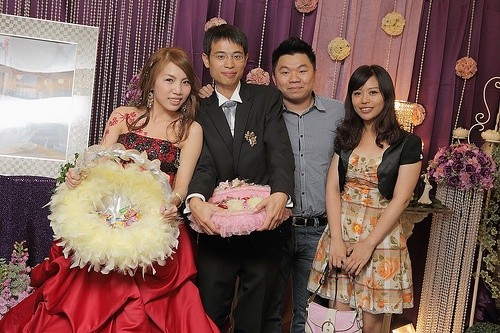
210,51,249,63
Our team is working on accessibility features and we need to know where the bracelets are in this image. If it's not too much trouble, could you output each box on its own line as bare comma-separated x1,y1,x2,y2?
176,192,182,208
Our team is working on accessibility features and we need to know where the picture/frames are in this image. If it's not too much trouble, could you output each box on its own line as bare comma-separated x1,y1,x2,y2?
0,14,99,179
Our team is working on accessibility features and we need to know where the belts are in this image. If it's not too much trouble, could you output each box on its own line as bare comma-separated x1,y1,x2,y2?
289,212,329,227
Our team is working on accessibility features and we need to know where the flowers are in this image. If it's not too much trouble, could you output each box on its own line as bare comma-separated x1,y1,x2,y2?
429,144,495,192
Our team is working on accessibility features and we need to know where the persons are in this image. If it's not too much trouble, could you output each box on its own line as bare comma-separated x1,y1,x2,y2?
200,37,345,333
0,48,220,333
306,65,423,333
181,26,296,333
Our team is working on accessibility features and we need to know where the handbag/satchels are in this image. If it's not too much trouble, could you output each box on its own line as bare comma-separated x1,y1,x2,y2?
304,262,363,333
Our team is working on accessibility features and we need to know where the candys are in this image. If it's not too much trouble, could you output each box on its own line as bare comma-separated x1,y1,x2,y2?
100,206,142,230
